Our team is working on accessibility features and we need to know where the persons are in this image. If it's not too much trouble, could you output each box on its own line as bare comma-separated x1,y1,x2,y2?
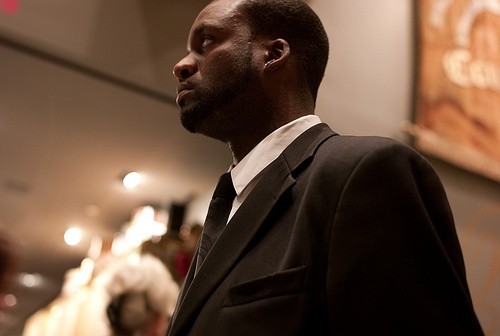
163,0,486,336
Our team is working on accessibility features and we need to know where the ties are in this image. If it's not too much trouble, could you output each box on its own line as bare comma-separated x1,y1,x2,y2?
193,171,238,275
